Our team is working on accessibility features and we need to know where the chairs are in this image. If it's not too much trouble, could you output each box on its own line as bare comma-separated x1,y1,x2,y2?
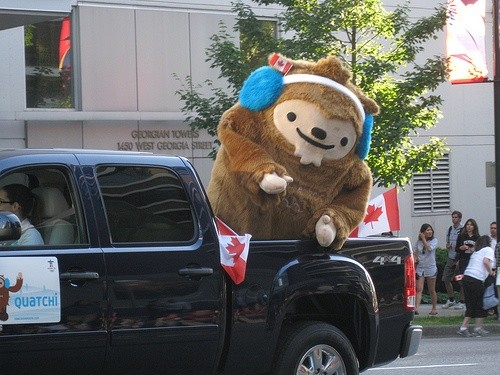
32,186,75,247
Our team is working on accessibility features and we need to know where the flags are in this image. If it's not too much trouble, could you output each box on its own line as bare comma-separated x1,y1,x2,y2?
270,55,292,73
216,217,252,284
348,188,400,238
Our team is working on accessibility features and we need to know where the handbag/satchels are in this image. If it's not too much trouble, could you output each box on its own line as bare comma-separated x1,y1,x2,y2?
482,276,499,310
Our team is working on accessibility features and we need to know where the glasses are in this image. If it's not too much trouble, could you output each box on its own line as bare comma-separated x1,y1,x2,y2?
0,200,14,206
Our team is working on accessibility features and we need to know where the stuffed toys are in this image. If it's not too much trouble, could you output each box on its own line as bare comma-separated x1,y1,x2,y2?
206,53,380,248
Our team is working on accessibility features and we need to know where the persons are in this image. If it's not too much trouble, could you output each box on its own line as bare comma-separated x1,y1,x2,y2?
413,224,437,315
442,211,464,309
454,235,496,337
481,222,498,319
0,183,43,246
455,218,480,274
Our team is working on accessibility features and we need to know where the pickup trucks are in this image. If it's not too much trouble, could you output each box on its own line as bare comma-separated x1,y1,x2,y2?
0,148,423,375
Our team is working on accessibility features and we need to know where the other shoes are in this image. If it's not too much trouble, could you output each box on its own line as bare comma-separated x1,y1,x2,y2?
472,327,490,336
457,328,473,337
428,310,438,316
442,299,457,308
415,310,419,315
448,302,465,309
